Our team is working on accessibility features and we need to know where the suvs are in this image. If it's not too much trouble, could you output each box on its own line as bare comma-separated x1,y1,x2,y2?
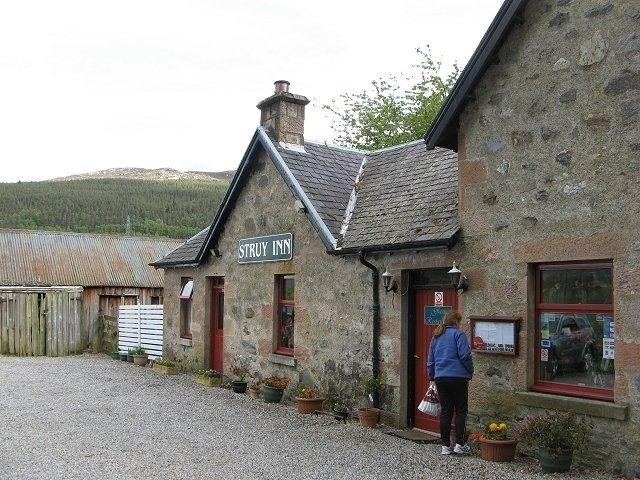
541,313,601,381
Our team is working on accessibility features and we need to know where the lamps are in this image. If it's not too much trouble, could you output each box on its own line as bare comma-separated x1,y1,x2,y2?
449,262,468,294
383,266,398,295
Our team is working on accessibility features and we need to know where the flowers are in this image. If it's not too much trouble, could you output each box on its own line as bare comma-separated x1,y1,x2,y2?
486,422,508,440
515,409,597,454
296,386,314,398
257,375,287,387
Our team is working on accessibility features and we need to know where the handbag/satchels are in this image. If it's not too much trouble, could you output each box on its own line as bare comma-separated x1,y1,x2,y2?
417,385,444,417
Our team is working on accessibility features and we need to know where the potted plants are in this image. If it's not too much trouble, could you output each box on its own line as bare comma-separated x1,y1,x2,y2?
103,315,178,374
196,358,260,398
333,403,349,420
353,376,383,427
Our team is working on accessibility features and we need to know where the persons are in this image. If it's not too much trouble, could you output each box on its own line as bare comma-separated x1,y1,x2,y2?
426,313,477,456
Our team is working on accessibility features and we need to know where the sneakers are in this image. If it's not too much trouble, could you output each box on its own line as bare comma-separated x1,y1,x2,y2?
453,443,471,454
441,444,453,455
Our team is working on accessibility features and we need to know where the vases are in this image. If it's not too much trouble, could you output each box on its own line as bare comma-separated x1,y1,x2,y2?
296,398,325,412
539,447,573,472
479,437,517,462
